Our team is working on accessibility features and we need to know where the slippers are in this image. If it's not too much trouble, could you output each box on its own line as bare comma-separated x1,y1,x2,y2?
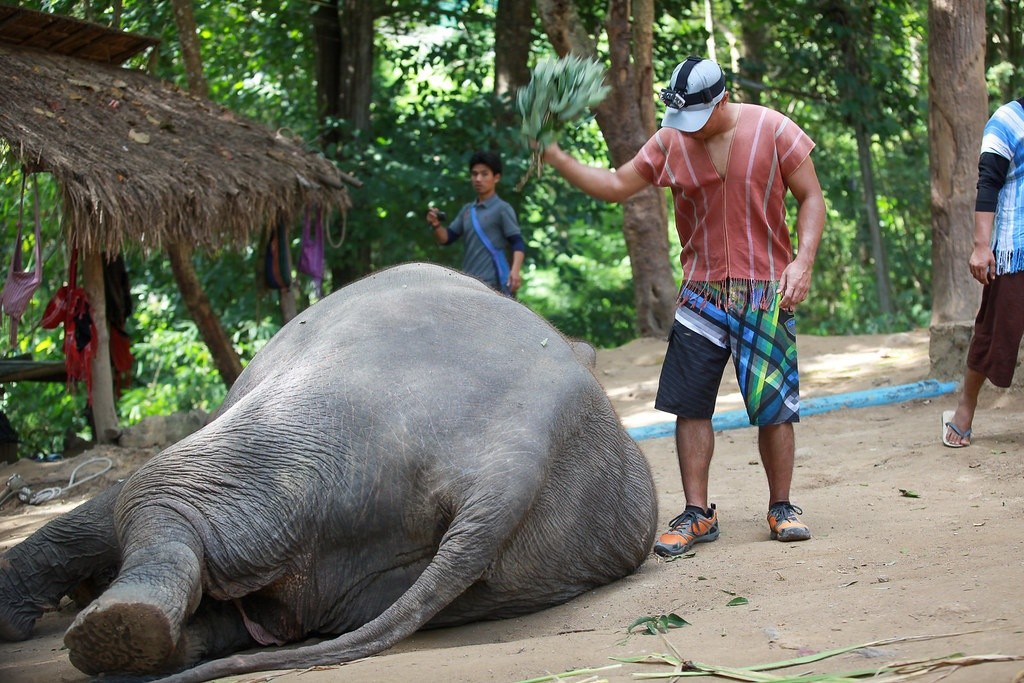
942,410,972,448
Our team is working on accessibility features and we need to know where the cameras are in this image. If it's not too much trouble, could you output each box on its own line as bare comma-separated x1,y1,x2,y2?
435,211,445,220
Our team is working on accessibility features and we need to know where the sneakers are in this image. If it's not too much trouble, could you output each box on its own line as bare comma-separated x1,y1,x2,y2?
653,503,719,557
766,501,810,543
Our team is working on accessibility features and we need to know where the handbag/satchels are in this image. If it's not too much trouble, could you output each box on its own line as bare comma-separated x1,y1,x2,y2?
493,250,513,298
2,235,42,344
297,237,324,278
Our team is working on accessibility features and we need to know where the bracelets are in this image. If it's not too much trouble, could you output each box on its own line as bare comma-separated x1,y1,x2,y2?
432,222,441,230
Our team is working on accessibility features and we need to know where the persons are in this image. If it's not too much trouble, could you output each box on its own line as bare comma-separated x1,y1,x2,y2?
427,152,526,297
942,96,1024,447
530,56,826,556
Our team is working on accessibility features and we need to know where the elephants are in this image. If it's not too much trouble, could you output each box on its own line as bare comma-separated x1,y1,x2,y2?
0,264,658,683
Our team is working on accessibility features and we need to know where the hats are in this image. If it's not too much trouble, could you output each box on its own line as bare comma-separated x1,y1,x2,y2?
660,57,725,133
